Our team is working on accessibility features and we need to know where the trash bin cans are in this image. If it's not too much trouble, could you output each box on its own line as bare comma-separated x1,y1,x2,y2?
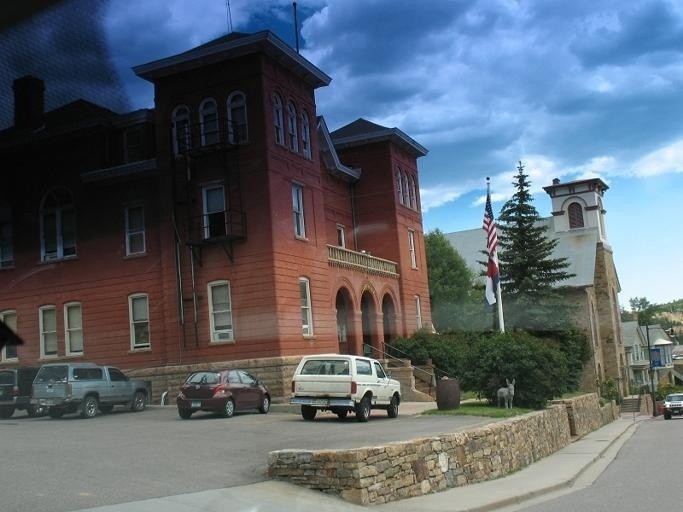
437,379,461,411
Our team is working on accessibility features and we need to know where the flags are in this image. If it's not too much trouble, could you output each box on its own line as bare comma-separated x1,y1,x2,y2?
481,187,499,306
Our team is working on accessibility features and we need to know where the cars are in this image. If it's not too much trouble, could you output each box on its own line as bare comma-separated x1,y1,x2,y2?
176,369,271,417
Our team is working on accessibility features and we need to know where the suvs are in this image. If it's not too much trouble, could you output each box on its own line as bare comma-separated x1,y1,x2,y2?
291,354,402,423
665,394,683,419
0,364,148,418
